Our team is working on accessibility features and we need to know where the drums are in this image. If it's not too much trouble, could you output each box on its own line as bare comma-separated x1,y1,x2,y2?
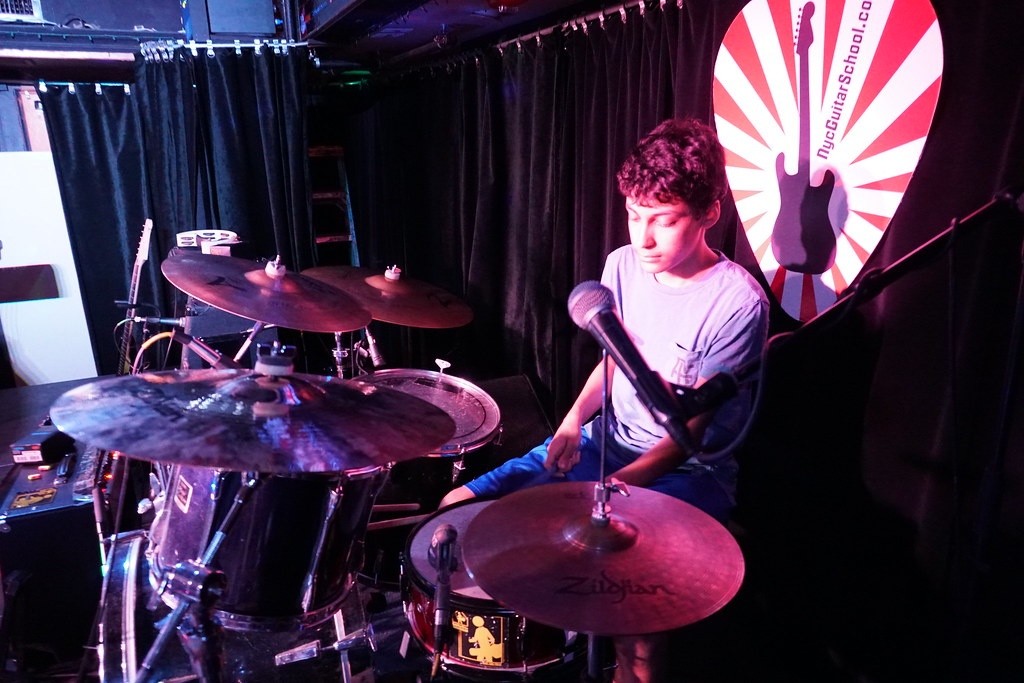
98,526,357,683
399,492,588,676
341,366,505,592
147,459,391,636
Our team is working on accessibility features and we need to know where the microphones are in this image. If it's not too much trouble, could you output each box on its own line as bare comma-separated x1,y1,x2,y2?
432,524,458,551
366,329,385,367
568,281,701,455
171,328,241,371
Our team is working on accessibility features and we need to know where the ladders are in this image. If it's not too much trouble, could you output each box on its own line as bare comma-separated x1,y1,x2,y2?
307,79,361,266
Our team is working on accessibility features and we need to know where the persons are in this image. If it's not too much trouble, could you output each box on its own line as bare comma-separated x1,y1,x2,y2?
438,119,770,683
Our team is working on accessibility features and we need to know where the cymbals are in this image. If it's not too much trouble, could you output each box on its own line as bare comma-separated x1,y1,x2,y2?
460,477,750,640
159,248,376,334
48,337,460,479
298,262,476,331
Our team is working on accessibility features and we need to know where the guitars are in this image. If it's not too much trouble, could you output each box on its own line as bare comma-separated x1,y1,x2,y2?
116,214,156,378
